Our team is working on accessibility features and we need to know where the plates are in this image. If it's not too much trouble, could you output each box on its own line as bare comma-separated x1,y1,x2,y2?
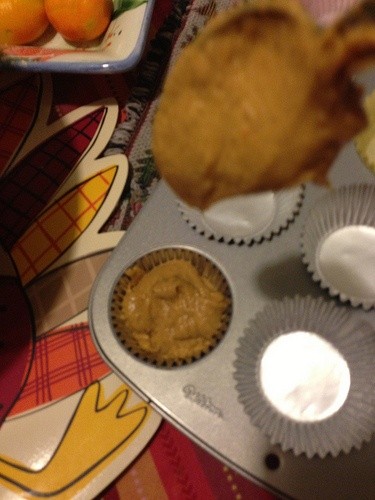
0,0,154,74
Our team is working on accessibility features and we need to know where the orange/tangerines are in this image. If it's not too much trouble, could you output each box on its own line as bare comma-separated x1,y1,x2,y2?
0,0,113,46
